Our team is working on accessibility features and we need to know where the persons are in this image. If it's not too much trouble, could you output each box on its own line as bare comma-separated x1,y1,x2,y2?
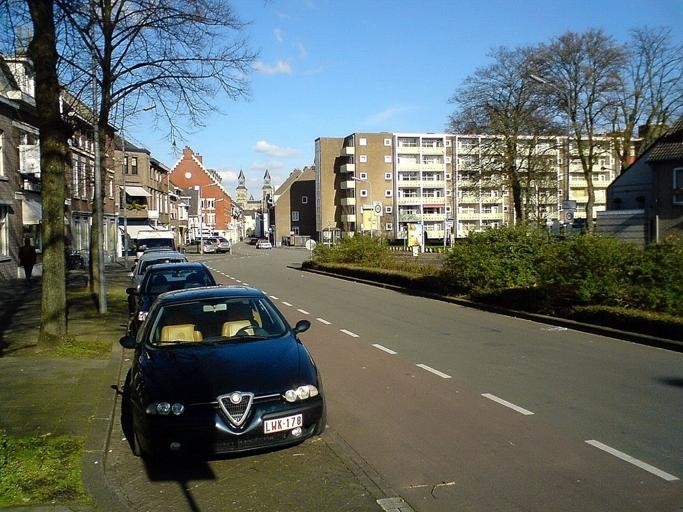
19,238,37,285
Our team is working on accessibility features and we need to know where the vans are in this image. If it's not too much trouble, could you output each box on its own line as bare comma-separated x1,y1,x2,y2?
134,229,176,261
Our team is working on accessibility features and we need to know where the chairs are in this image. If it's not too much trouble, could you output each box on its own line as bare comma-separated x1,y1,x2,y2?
220,319,255,337
161,324,203,345
150,276,171,293
184,273,203,289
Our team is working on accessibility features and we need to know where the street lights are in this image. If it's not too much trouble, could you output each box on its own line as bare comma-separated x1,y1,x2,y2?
95,101,158,128
350,176,373,241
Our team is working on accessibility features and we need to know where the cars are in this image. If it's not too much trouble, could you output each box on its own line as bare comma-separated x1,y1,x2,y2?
123,260,214,335
131,251,192,287
249,235,259,245
125,285,325,462
177,239,216,254
255,238,272,249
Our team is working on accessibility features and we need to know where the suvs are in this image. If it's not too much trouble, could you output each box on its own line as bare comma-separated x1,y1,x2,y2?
208,236,231,253
